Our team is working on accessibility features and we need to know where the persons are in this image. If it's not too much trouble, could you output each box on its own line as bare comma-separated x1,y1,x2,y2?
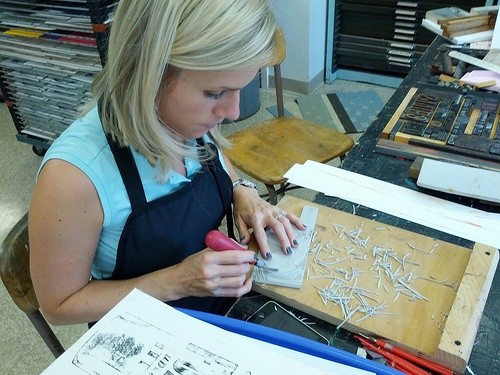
29,0,307,331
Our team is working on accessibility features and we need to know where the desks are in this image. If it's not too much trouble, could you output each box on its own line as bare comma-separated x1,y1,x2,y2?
222,35,500,375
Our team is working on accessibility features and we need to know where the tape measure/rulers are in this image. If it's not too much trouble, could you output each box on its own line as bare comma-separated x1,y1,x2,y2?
447,51,500,74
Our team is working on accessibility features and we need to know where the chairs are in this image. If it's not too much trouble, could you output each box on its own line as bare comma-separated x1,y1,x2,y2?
218,23,354,205
0,211,76,359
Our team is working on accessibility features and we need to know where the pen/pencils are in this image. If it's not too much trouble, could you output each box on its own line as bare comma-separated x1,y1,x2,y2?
354,332,459,375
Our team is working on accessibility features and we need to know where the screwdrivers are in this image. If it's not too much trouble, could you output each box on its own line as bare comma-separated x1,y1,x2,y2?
444,41,491,50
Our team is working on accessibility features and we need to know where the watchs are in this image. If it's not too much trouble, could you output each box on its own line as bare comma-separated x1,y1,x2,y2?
232,178,258,190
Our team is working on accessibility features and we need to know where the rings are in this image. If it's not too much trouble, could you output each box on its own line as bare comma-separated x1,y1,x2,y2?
275,214,284,219
